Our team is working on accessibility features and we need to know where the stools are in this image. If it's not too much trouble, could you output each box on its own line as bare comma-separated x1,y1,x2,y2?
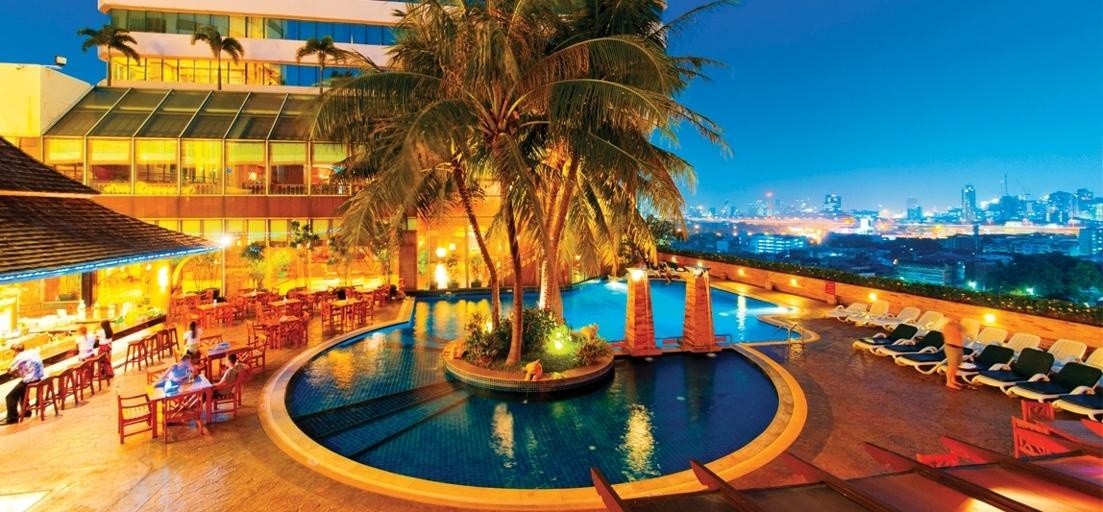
20,352,110,422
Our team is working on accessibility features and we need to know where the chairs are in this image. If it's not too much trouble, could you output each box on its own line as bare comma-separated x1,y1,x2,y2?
852,322,920,356
1009,361,1102,404
959,318,981,339
894,343,975,375
1052,387,1103,422
880,330,946,366
644,259,712,278
976,327,1008,346
972,346,1055,395
875,307,921,332
1086,347,1103,369
1007,332,1041,353
937,343,1015,384
906,310,944,337
849,300,890,328
827,302,868,323
118,284,393,444
1047,339,1088,364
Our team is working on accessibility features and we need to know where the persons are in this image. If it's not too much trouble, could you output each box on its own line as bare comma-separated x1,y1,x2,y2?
183,321,203,374
332,290,350,307
943,315,965,391
0,344,44,425
661,264,673,285
520,358,543,382
162,354,193,385
75,327,95,382
202,353,250,402
96,320,114,379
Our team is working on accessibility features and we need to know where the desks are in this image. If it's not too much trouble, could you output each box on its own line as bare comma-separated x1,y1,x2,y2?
144,374,214,439
197,302,234,330
267,315,300,349
267,298,302,315
199,342,253,383
330,298,361,327
